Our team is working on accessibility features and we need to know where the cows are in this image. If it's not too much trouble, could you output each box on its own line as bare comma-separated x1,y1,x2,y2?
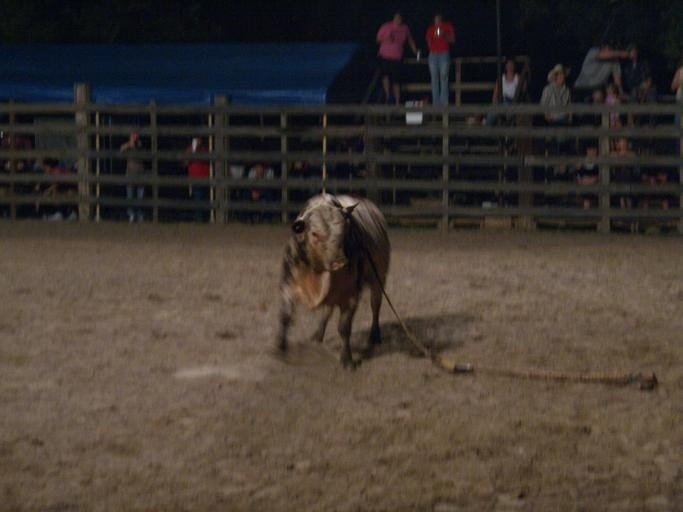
273,192,392,372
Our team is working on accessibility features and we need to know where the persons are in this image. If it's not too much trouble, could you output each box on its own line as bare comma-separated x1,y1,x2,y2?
375,14,418,105
121,133,149,221
448,41,683,206
248,164,277,203
286,161,311,204
184,137,211,222
426,14,457,105
15,157,79,222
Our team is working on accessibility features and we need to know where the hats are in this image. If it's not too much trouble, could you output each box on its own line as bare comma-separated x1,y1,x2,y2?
547,63,570,83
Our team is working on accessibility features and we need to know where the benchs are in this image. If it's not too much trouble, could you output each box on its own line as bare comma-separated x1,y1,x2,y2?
354,54,683,164
